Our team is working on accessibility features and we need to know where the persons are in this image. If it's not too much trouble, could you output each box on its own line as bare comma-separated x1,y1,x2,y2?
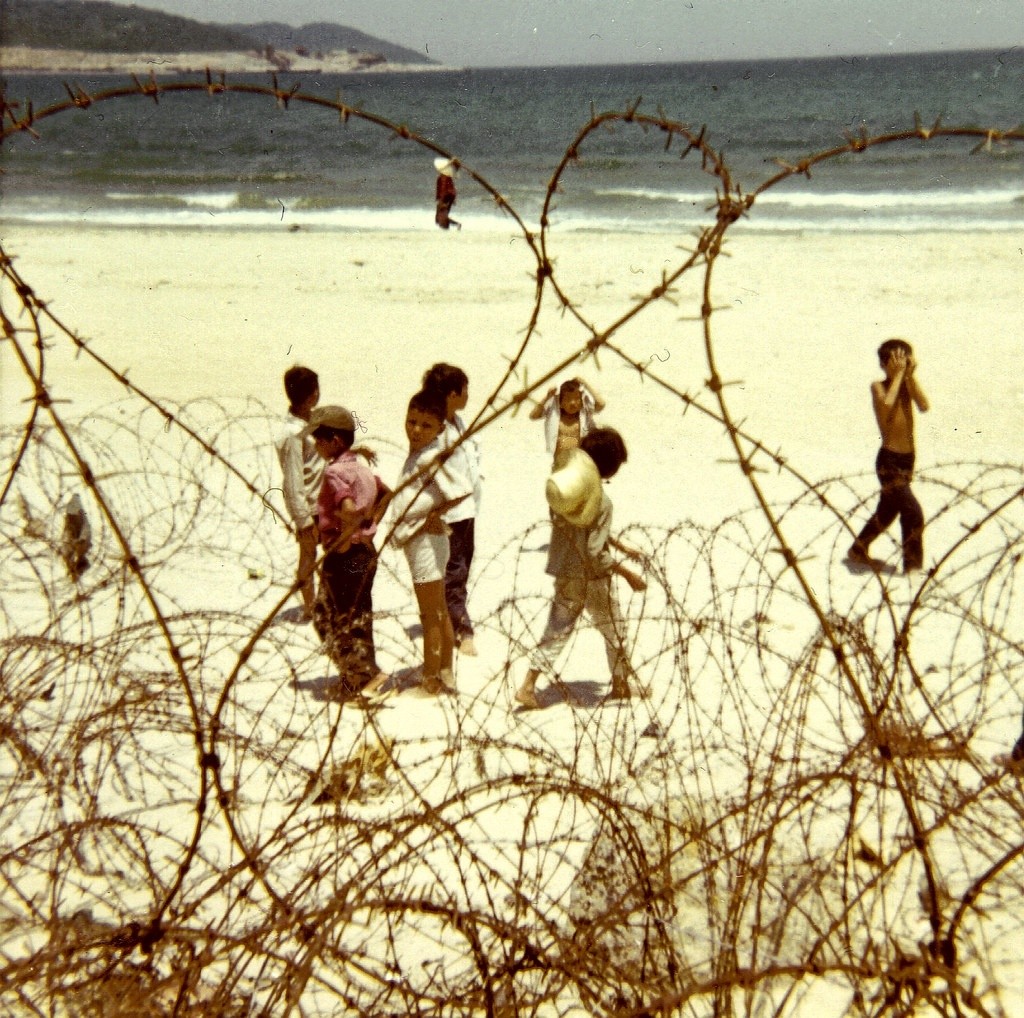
272,365,392,695
387,363,482,698
514,376,654,709
847,339,928,573
433,155,462,230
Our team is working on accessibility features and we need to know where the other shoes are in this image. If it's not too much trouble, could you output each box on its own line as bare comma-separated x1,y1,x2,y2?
458,223,462,231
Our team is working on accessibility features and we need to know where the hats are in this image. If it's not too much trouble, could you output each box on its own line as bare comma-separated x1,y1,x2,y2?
545,448,601,526
434,157,456,178
296,406,355,438
